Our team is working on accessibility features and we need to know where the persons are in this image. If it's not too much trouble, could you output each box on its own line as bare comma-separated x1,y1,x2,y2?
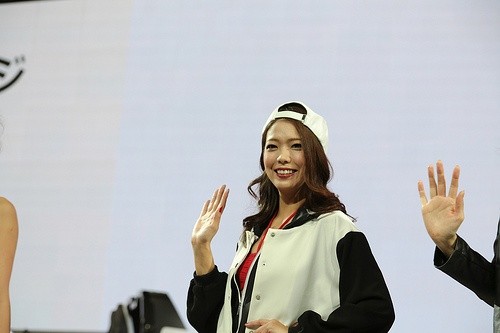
0,196,19,333
185,100,396,333
416,159,500,333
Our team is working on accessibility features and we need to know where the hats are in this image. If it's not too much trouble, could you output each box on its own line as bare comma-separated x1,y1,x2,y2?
261,101,328,153
